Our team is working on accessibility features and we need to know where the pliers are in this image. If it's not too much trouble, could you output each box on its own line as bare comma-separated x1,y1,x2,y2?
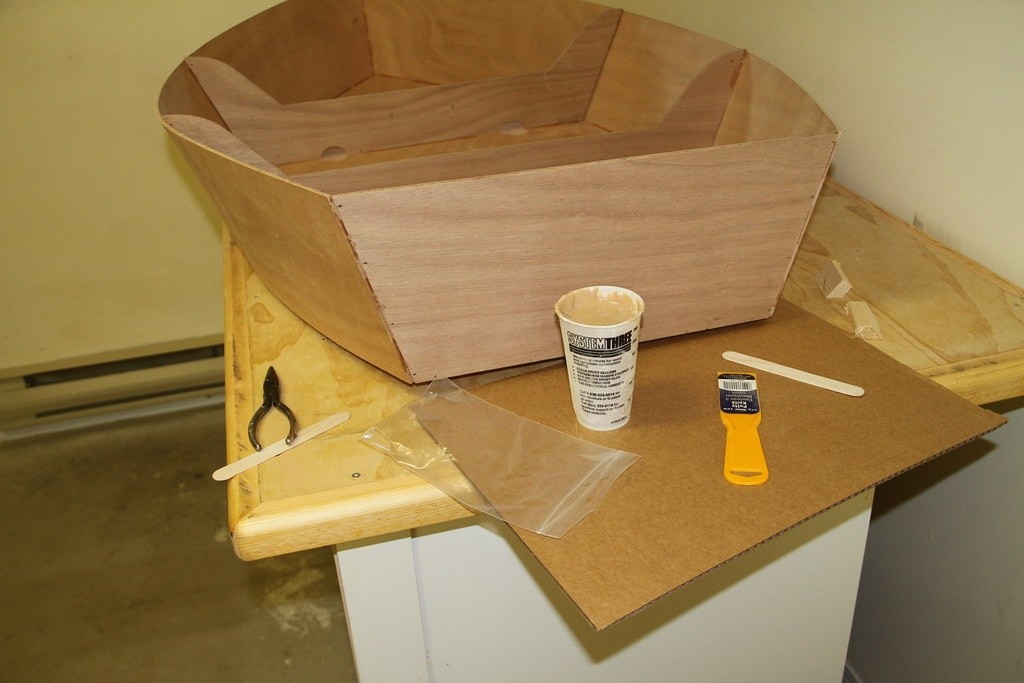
248,365,299,452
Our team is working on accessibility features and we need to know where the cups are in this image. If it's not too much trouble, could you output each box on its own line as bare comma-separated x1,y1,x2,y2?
554,285,646,431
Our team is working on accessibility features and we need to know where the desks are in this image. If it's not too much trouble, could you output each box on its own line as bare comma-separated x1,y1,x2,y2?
219,176,1024,683
